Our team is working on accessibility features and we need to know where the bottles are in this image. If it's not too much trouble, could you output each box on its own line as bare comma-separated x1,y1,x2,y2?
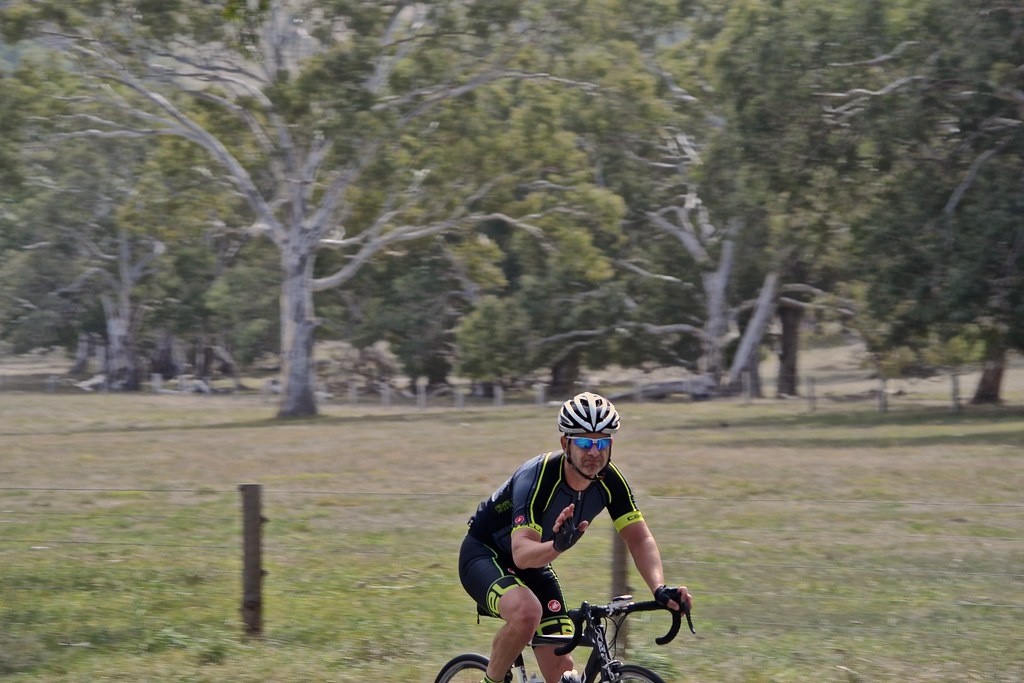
558,669,581,683
527,672,544,683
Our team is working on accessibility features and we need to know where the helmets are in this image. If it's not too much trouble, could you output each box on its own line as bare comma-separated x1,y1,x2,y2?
557,391,621,434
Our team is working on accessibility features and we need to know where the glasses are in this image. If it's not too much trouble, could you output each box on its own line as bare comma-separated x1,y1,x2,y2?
565,435,613,451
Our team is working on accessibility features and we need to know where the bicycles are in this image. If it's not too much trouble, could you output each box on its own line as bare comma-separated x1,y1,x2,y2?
438,589,695,683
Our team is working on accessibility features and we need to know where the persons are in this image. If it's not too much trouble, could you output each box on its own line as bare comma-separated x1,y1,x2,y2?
458,392,693,683
